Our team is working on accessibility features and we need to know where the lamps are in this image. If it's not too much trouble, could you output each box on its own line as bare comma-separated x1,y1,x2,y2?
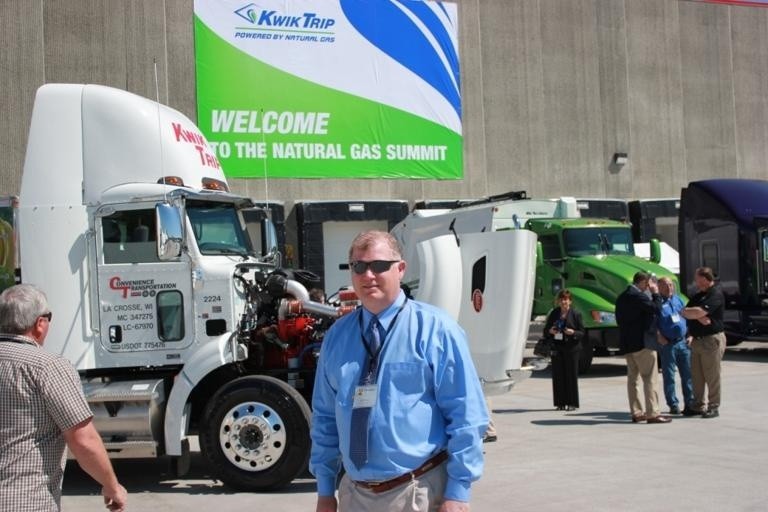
614,152,627,166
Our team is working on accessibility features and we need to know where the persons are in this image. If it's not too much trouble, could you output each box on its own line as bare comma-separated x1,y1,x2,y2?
648,275,694,417
541,288,588,413
308,230,491,512
678,265,727,418
613,269,673,425
1,283,129,512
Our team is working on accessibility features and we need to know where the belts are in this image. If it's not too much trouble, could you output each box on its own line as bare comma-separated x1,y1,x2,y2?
352,451,447,494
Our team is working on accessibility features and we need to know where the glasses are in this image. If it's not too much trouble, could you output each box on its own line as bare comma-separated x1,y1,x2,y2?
349,261,398,273
41,312,51,321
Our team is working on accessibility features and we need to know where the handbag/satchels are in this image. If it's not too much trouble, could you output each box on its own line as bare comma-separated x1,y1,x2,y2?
532,339,554,357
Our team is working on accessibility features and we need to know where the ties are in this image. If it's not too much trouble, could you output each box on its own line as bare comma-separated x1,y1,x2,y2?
349,317,381,471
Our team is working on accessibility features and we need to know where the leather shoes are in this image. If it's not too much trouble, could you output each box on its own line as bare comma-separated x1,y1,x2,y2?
557,404,575,411
633,405,719,423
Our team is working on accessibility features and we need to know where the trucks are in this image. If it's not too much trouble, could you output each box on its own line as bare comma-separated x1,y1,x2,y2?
629,178,767,352
0,82,537,491
390,197,686,360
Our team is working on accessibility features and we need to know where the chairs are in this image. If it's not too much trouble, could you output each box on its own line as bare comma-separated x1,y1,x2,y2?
134,226,149,242
